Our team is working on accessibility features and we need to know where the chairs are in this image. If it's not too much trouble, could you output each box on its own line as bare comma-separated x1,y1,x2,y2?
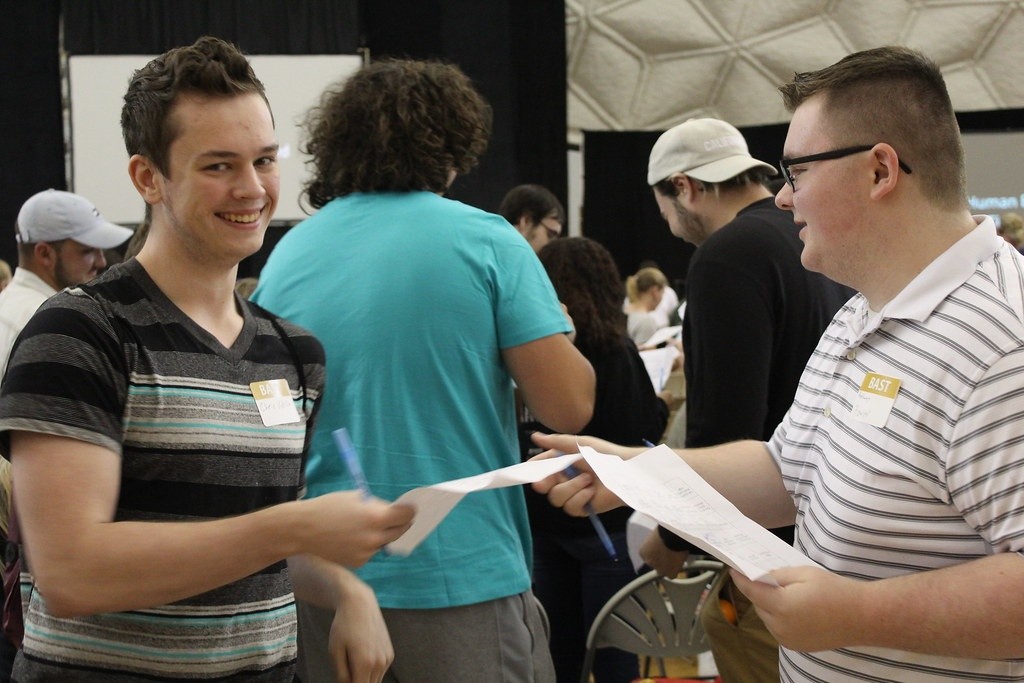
585,559,724,683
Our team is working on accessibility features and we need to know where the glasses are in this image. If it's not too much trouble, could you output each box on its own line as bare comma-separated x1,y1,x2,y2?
780,145,912,192
540,223,558,242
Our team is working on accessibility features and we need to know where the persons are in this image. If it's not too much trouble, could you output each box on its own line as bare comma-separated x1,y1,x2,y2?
249,57,598,683
495,119,1024,683
0,35,416,683
530,46,1024,683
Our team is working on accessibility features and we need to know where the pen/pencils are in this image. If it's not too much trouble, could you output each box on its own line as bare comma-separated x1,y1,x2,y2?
565,466,619,563
332,427,373,499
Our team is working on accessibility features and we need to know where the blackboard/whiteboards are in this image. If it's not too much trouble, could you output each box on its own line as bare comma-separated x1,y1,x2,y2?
62,48,373,231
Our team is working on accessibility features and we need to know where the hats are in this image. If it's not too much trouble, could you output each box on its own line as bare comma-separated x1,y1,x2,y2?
15,188,135,250
647,118,778,185
